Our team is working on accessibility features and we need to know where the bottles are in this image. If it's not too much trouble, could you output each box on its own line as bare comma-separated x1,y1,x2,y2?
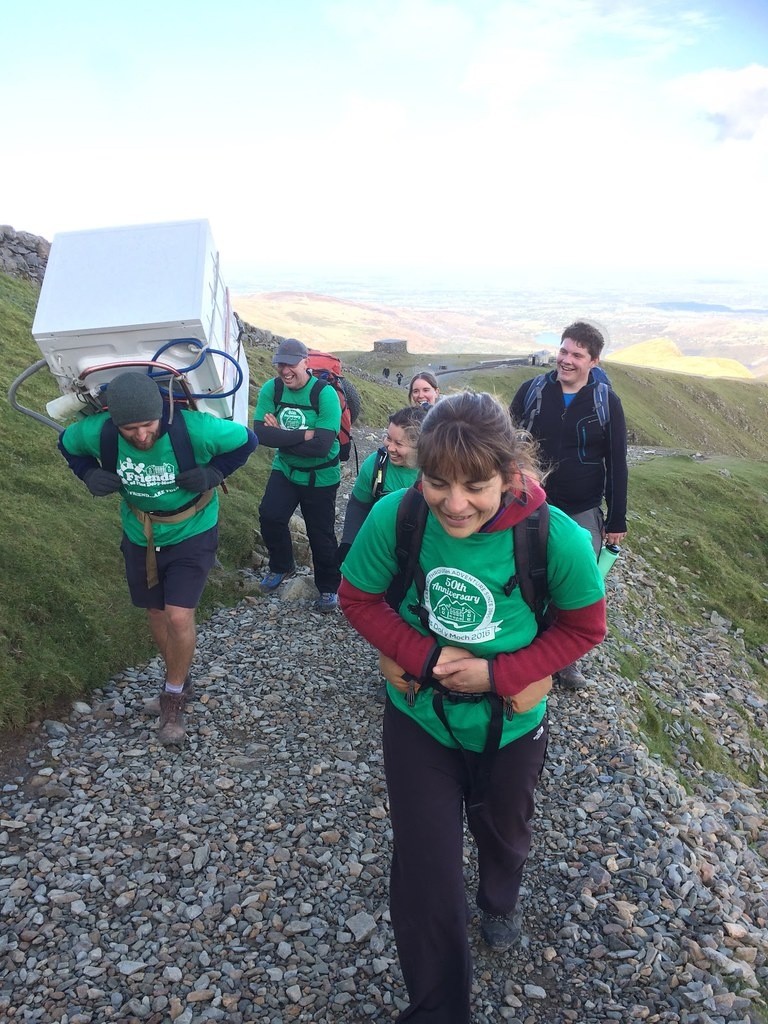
596,544,620,578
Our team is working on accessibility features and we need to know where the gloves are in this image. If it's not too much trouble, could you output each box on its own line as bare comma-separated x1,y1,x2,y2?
334,543,351,580
175,465,224,493
83,468,121,496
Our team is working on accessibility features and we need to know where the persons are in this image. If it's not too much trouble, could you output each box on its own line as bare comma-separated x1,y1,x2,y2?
506,322,628,694
58,372,258,743
253,338,342,613
396,372,403,385
338,371,440,572
337,378,606,1024
382,367,390,379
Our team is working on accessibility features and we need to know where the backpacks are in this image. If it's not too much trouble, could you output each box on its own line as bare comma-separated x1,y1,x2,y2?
517,366,614,455
274,348,360,473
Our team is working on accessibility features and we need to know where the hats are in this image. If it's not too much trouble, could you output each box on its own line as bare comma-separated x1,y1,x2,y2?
272,339,307,365
105,371,163,427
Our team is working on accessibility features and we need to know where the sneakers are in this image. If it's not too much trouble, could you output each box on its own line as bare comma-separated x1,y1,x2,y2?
143,672,196,716
158,690,188,745
480,896,523,952
315,593,337,612
258,563,297,593
558,659,587,688
